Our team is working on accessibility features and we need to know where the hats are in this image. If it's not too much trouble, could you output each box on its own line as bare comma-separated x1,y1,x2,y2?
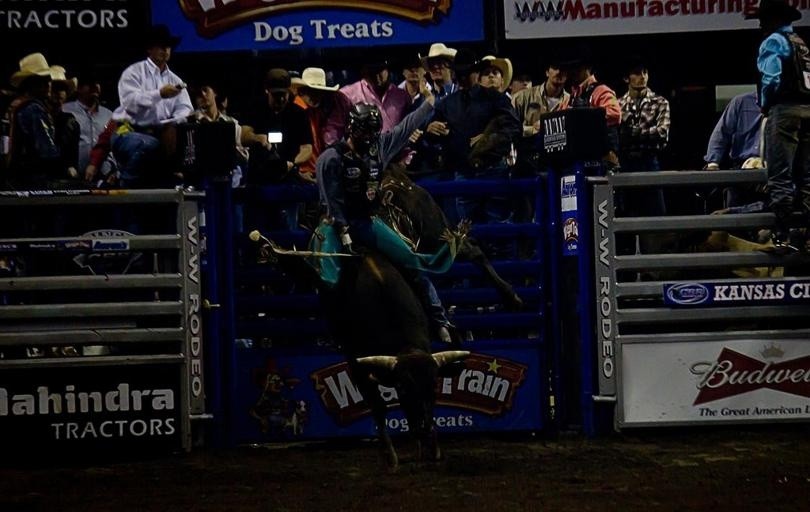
137,23,180,48
743,0,802,22
289,66,341,93
9,50,57,88
50,63,79,96
422,42,459,60
475,54,515,94
263,66,293,94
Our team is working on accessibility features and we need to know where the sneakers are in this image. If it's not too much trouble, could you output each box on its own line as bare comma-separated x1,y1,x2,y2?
434,325,453,344
766,231,793,251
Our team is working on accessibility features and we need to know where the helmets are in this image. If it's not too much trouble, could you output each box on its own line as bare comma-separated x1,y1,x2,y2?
344,101,387,153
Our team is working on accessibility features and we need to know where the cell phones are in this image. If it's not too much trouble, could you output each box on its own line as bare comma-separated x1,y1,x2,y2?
176,83,187,89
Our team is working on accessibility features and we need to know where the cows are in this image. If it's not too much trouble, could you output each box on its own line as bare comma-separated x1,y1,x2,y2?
319,170,523,476
